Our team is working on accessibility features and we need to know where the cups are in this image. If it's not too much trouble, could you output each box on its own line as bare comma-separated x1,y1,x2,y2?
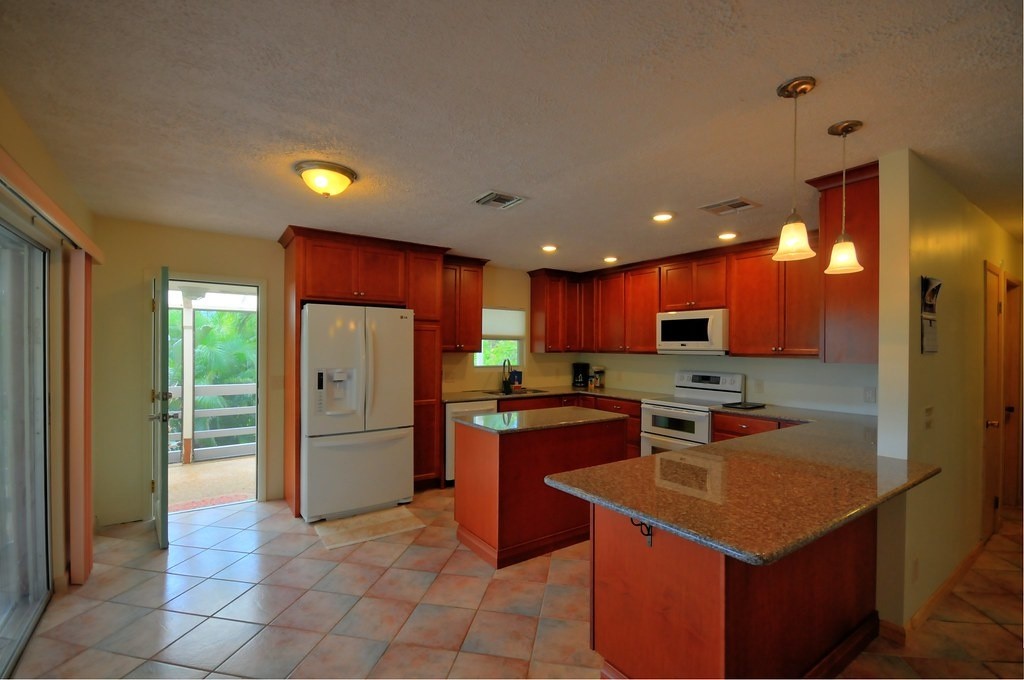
510,384,522,394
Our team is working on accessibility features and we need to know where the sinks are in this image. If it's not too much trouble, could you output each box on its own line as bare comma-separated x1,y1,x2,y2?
483,390,549,395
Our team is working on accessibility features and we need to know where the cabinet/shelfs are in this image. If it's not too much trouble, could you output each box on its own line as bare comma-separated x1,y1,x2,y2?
727,233,819,357
443,259,482,353
530,264,661,355
406,250,443,324
561,394,641,459
661,253,727,312
284,236,406,306
819,175,880,364
414,326,442,490
711,412,779,442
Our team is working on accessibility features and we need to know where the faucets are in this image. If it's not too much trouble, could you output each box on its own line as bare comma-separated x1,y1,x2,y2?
502,358,512,380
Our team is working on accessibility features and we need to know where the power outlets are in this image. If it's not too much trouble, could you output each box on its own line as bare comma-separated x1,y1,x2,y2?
863,386,876,403
616,370,624,382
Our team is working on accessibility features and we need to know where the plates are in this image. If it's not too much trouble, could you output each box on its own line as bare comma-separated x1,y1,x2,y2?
721,402,766,409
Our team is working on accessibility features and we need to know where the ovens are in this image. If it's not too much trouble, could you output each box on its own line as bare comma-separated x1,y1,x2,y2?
640,404,712,457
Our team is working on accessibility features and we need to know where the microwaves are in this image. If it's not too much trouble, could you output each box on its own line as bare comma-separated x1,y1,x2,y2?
656,308,729,356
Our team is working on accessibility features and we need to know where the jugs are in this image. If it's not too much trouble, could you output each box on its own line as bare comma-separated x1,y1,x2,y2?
577,373,584,381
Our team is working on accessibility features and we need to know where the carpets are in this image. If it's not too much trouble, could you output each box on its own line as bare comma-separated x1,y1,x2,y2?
313,504,425,551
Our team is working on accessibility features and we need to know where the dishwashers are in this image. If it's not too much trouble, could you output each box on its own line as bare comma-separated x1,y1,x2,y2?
445,401,497,482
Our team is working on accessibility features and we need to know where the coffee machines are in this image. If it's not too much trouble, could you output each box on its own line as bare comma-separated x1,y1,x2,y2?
572,362,589,388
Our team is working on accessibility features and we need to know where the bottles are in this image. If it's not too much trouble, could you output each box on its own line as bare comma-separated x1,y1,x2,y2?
588,370,605,388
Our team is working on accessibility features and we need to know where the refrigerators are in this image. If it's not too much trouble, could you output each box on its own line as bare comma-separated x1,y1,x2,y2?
299,302,415,523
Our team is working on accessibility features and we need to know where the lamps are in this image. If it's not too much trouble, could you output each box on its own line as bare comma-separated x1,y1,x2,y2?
294,161,357,198
772,75,816,261
823,121,864,274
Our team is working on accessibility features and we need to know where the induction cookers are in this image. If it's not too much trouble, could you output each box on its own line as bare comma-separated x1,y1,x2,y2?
641,371,745,413
